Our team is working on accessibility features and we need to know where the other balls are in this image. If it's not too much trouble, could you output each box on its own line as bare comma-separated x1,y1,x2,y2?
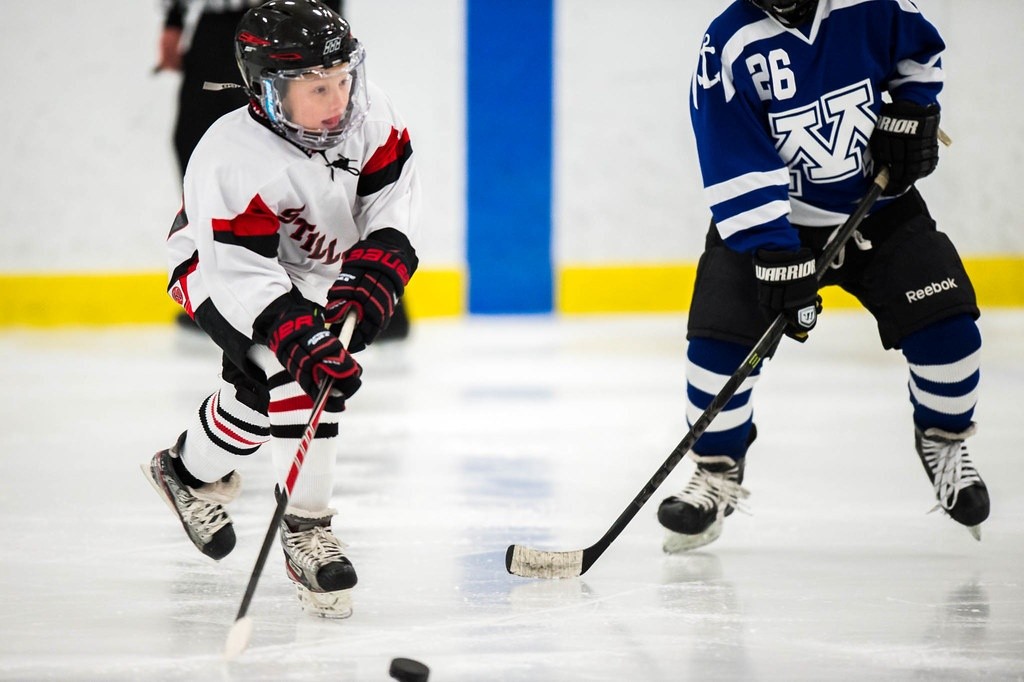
387,657,432,682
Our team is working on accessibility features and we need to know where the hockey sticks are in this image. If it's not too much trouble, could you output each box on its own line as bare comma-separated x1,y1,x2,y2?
222,311,358,655
505,171,888,581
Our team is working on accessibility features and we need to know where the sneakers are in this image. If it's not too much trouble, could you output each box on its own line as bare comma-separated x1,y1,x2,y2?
914,422,991,542
141,431,236,563
279,514,358,620
657,448,752,555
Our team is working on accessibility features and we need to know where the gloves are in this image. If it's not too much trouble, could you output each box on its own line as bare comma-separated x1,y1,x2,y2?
868,102,940,199
322,269,400,353
750,253,820,336
271,303,363,412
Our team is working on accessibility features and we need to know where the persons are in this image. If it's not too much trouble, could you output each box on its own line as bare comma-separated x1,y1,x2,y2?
140,0,420,619
159,0,410,345
657,0,989,552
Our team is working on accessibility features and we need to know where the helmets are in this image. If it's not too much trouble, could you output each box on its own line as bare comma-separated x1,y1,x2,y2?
234,1,364,98
